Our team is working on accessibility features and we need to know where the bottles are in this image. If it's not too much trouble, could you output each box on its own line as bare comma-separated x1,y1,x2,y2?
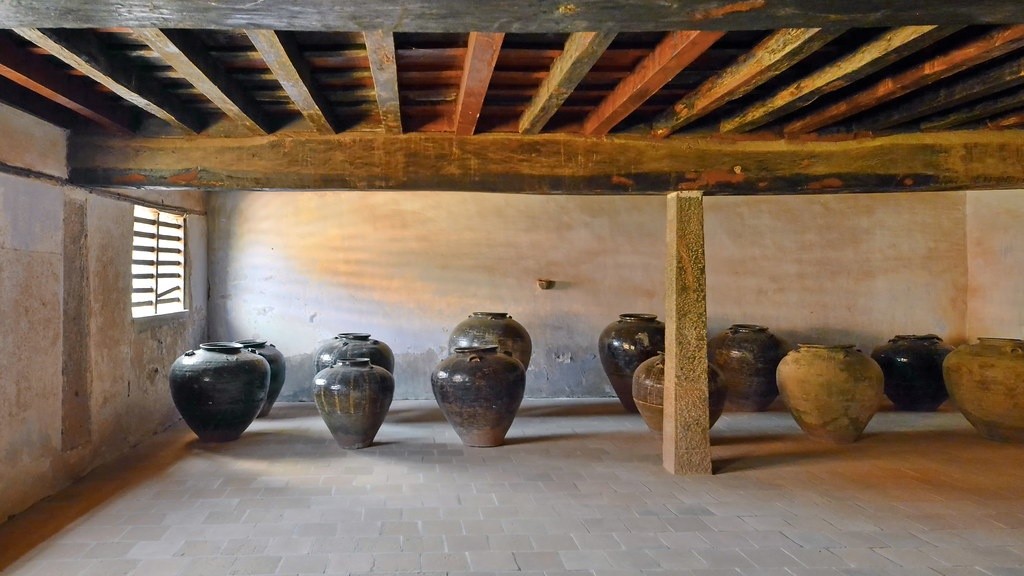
312,333,394,374
777,343,884,443
168,342,270,443
632,351,726,448
708,323,787,412
942,338,1024,444
312,358,394,449
448,312,531,370
238,341,286,418
872,335,957,411
431,345,526,447
599,314,665,414
